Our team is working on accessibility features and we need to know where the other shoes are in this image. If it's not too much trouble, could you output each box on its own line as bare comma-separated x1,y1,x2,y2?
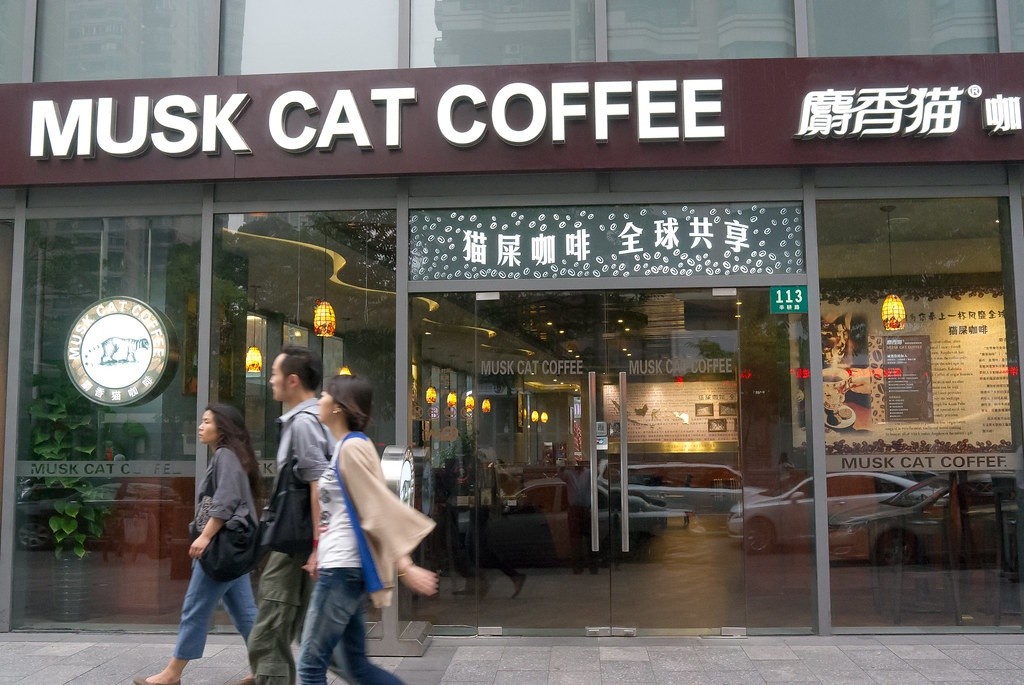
132,677,181,685
231,678,255,685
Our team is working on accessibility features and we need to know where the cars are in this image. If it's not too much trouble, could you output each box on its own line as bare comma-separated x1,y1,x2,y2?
7,482,185,550
454,460,1018,571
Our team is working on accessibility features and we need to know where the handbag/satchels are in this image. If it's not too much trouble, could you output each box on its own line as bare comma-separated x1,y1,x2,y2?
260,410,331,557
191,492,261,582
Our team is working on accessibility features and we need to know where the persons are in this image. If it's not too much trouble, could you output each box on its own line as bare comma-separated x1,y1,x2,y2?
134,404,262,685
299,375,439,685
555,451,599,575
449,429,527,601
246,346,356,685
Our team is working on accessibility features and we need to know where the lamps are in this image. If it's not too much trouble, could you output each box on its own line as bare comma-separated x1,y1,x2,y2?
246,286,262,374
426,347,547,424
314,216,335,336
338,322,351,377
880,207,905,332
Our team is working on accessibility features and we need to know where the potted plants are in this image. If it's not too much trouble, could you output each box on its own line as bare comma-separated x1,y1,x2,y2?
18,370,178,622
